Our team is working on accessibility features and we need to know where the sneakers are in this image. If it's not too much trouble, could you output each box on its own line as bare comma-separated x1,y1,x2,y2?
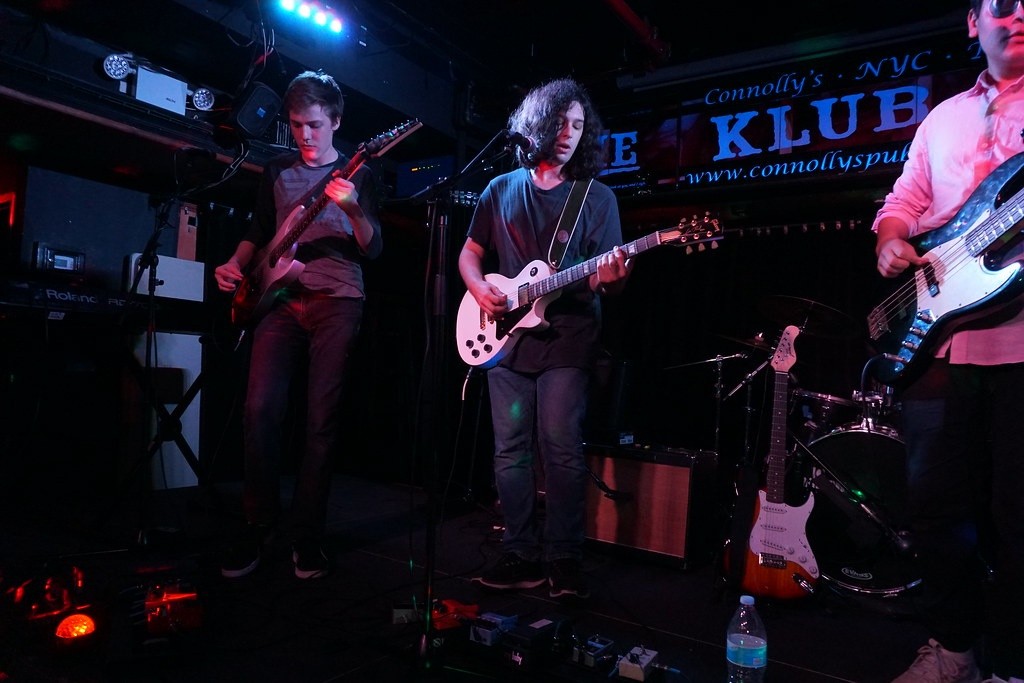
890,639,981,683
480,548,546,589
220,532,272,578
292,541,330,580
546,557,591,600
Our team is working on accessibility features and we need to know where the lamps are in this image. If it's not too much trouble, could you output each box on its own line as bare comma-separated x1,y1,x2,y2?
102,52,215,119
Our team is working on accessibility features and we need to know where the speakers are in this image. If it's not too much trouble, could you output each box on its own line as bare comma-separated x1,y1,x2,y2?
232,82,284,137
580,441,719,570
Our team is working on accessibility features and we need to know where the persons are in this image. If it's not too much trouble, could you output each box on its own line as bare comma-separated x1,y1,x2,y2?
460,80,634,597
214,70,381,578
870,0,1024,683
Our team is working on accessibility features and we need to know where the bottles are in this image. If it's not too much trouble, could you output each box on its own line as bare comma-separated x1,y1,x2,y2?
727,595,768,683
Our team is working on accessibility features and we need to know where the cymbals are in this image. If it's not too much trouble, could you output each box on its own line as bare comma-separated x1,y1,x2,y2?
712,316,805,354
743,291,850,325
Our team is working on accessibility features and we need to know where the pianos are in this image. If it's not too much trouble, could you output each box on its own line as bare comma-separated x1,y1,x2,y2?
0,279,212,504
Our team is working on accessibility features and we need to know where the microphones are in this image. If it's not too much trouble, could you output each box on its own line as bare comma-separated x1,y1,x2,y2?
890,532,912,553
505,132,536,154
604,492,633,503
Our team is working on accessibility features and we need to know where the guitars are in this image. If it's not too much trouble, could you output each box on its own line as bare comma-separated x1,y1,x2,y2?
722,324,823,600
210,115,425,362
866,151,1024,392
455,209,728,371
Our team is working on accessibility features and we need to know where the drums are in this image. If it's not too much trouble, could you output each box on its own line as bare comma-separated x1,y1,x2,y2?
780,420,928,595
762,385,863,447
